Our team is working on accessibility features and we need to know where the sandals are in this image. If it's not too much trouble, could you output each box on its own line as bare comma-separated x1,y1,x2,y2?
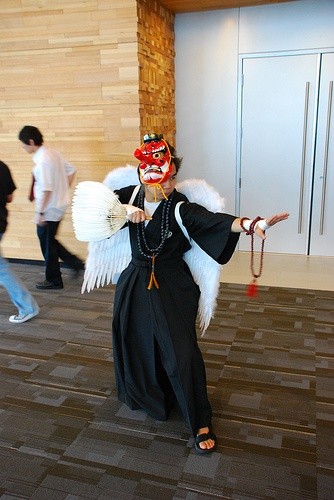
194,425,218,453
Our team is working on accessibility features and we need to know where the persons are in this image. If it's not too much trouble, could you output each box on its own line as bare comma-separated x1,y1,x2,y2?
19,125,83,289
72,134,289,455
0,160,40,323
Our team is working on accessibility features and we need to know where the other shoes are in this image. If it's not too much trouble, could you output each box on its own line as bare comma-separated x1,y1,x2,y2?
36,279,64,289
8,307,40,324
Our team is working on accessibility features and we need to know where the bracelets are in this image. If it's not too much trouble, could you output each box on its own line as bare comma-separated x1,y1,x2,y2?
39,212,44,216
240,217,265,299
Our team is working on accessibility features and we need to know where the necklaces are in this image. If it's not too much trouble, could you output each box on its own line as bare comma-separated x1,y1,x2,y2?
137,191,173,290
